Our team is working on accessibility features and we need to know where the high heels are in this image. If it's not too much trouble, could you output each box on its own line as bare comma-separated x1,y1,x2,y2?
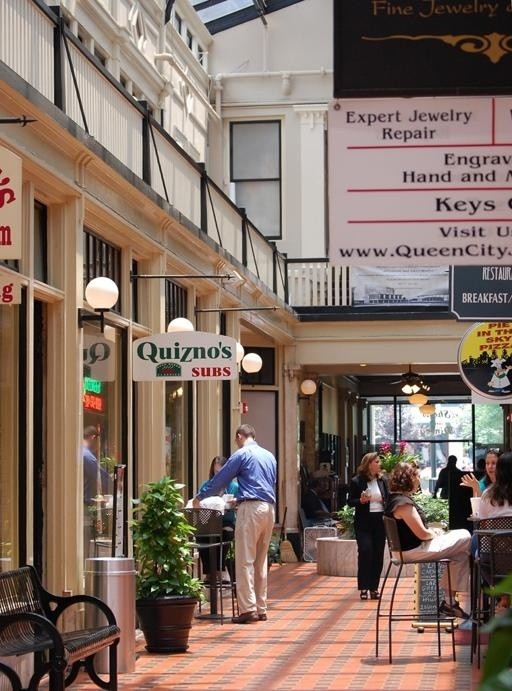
370,590,380,600
359,590,369,601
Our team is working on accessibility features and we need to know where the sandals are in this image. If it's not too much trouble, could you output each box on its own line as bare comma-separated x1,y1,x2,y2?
436,600,470,621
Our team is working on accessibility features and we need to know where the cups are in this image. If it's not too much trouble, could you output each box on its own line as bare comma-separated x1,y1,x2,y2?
470,498,480,516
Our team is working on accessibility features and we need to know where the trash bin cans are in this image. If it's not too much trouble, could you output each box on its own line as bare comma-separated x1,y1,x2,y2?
84,558,134,674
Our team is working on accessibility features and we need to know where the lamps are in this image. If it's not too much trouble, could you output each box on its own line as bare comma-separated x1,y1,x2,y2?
298,380,317,400
78,276,119,333
168,317,193,332
397,364,436,415
236,342,263,384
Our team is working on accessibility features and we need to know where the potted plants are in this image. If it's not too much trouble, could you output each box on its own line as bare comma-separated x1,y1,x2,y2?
125,475,209,653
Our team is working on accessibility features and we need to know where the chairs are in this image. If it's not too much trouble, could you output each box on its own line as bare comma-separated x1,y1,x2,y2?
298,509,338,527
0,565,122,691
376,515,512,669
172,508,233,625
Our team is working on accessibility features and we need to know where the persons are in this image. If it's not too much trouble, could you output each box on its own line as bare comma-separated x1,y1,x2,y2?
433,456,464,499
459,451,512,617
302,477,338,527
477,459,486,472
84,425,114,559
195,457,238,583
385,461,472,619
477,448,503,493
347,452,390,600
192,424,278,623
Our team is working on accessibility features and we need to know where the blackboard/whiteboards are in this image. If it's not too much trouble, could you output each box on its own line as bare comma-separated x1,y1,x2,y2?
411,560,459,627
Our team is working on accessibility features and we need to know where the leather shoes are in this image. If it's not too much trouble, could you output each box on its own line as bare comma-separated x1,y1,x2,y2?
232,612,260,623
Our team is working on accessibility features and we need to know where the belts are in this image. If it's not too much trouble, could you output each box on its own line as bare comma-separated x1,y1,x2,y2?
237,499,268,504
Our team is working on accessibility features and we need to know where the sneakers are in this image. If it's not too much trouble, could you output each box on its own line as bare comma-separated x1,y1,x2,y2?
257,615,268,621
